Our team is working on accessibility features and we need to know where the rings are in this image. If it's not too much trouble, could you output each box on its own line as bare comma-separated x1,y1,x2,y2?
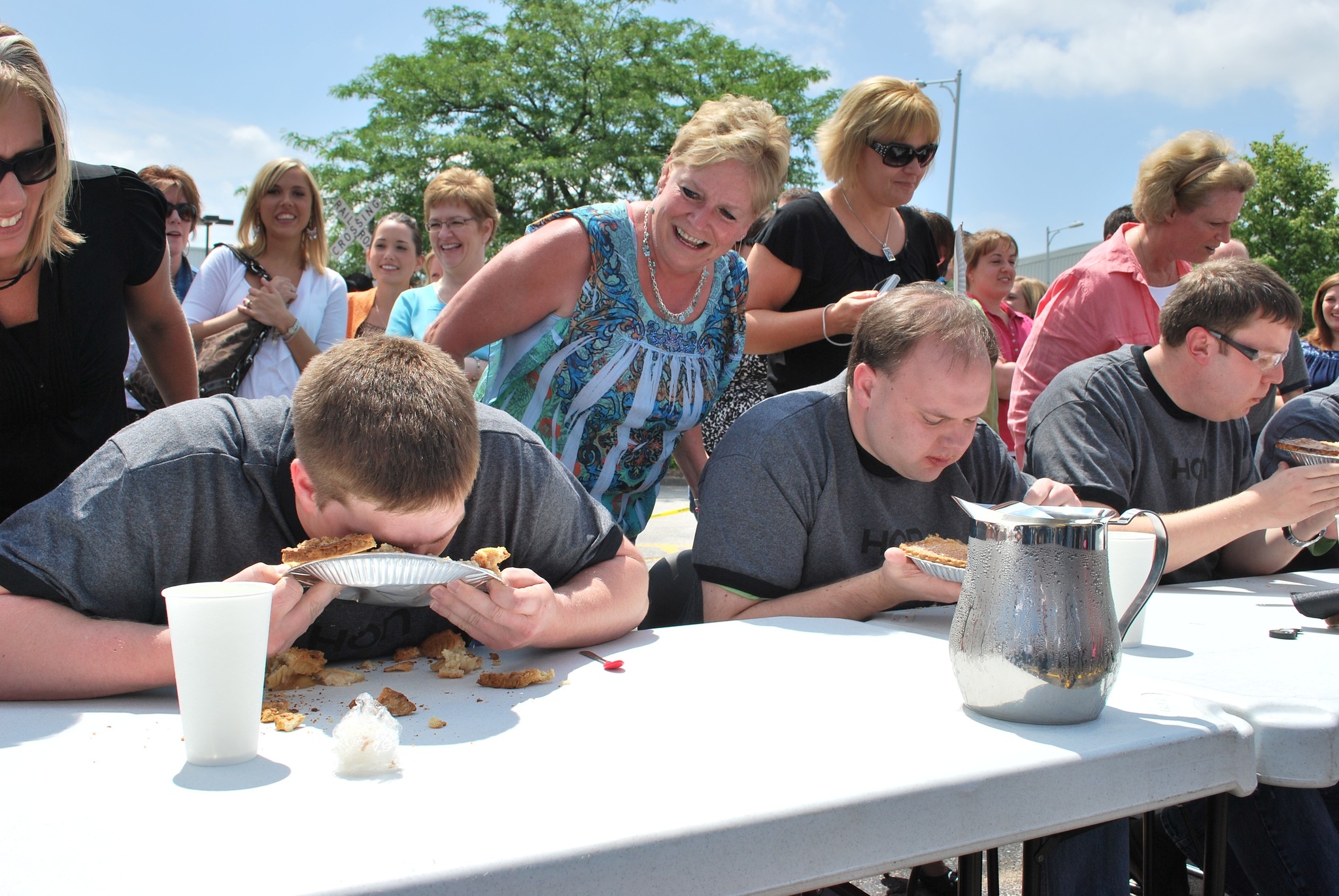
247,301,251,308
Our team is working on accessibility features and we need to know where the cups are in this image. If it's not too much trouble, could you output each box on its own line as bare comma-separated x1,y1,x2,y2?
161,580,274,763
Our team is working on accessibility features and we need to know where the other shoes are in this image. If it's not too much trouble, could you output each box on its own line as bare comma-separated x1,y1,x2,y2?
909,866,958,896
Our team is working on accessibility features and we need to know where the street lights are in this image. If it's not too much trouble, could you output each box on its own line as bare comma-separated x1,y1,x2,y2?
1045,222,1085,284
200,214,234,258
907,69,964,224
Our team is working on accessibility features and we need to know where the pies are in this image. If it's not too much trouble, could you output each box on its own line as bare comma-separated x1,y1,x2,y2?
257,532,554,730
898,535,969,570
1276,438,1339,458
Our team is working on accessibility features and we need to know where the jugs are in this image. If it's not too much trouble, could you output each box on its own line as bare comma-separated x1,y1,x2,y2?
949,494,1170,726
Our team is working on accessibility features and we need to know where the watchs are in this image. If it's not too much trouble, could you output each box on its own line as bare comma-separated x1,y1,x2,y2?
281,318,300,339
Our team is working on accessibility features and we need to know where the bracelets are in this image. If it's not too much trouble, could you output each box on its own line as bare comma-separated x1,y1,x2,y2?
282,322,301,343
1281,524,1327,548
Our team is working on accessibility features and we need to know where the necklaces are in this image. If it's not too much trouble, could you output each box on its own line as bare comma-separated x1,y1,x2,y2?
1137,225,1174,287
840,180,896,261
0,254,38,292
642,202,710,323
374,301,388,329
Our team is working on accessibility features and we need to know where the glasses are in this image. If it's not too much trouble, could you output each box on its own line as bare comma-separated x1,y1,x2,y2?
425,217,476,233
1204,330,1290,371
0,142,65,186
864,137,938,168
167,199,198,221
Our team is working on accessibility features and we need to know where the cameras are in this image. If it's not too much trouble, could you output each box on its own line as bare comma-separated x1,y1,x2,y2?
871,275,900,298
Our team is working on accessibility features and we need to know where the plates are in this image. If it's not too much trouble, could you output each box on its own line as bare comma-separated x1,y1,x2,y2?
281,552,505,608
905,554,966,583
1275,445,1339,465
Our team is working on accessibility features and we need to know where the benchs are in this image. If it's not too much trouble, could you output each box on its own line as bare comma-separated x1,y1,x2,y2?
0,566,1339,896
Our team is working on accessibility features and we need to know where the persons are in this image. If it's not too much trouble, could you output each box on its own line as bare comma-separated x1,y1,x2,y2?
0,334,651,701
742,75,944,398
1024,254,1339,586
0,25,200,524
690,281,1083,625
1007,130,1258,467
126,158,501,419
699,190,1047,460
422,91,790,546
1103,207,1339,570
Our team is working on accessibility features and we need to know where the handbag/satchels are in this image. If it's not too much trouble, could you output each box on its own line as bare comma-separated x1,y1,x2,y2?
125,243,271,414
